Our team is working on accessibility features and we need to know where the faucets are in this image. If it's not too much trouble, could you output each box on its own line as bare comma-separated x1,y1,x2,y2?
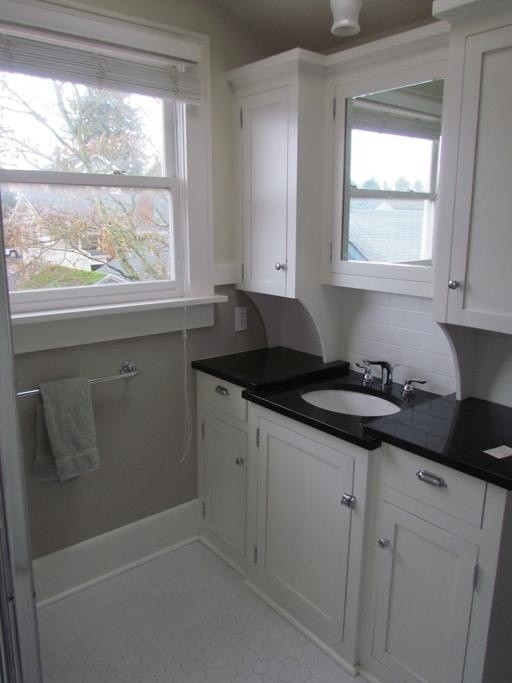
363,359,393,390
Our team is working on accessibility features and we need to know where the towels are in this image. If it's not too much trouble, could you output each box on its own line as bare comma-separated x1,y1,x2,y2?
31,375,98,485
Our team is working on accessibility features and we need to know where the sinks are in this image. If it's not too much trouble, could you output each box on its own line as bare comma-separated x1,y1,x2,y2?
298,384,406,416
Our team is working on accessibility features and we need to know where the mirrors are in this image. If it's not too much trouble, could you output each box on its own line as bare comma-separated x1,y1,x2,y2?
338,79,446,269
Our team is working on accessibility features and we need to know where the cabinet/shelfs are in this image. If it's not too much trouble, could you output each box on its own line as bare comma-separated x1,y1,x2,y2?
244,399,369,677
194,371,253,577
227,46,324,299
430,0,512,336
359,441,512,683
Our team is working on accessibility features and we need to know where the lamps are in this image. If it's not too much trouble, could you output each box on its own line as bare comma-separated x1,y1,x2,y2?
328,0,363,38
